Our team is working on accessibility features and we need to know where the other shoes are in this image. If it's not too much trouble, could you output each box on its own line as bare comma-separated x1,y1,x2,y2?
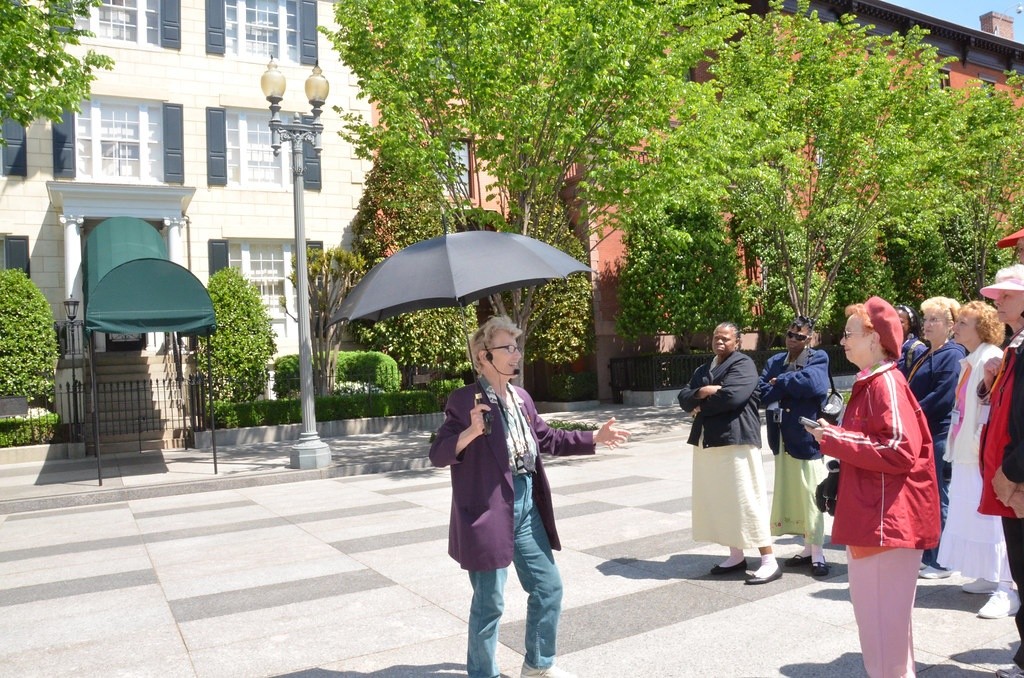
918,565,952,579
920,562,927,570
997,665,1024,678
746,564,784,585
711,558,749,575
811,555,828,576
785,554,812,567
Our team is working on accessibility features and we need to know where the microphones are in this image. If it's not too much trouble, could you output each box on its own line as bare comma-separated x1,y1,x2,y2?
490,362,520,375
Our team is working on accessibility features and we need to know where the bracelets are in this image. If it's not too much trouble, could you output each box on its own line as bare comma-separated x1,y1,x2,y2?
694,408,698,412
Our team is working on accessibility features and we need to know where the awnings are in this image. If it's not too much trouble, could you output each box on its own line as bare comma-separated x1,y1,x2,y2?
81,216,216,486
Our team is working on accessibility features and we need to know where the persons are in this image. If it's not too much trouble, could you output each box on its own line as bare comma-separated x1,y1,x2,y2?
431,316,631,678
894,305,926,375
804,298,940,678
760,317,829,575
976,231,1024,678
909,297,967,579
937,300,1021,619
679,324,782,585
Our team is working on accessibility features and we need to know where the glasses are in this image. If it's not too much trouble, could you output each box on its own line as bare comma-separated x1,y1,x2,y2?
787,329,812,342
844,330,869,339
482,345,522,354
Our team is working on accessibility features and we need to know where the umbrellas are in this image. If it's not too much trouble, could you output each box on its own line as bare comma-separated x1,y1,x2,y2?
325,208,598,436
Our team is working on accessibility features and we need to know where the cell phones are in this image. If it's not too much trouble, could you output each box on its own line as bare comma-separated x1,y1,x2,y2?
798,417,821,429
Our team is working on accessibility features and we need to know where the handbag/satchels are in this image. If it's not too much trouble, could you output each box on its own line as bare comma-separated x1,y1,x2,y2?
816,460,840,517
814,367,844,427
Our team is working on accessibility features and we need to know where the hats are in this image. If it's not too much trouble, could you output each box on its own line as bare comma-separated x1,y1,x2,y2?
980,279,1024,299
997,229,1024,249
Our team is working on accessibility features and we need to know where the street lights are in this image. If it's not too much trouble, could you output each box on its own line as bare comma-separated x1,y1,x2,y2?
262,55,337,471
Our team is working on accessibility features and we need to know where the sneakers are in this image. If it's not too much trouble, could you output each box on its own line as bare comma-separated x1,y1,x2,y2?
978,587,1020,619
962,577,999,593
